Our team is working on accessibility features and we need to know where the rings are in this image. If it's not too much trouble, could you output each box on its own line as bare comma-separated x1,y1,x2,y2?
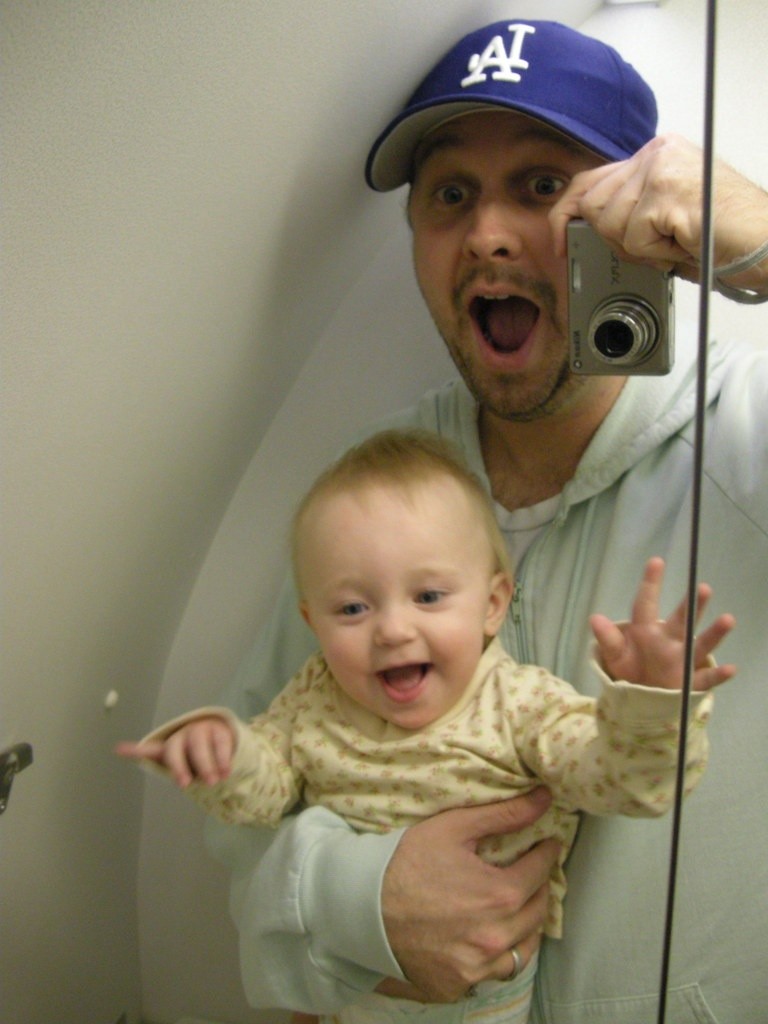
496,947,521,983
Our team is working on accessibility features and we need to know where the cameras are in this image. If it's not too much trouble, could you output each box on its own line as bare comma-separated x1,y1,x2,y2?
567,220,676,376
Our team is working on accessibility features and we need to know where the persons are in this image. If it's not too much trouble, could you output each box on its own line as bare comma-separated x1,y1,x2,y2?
200,16,767,1024
113,427,736,1023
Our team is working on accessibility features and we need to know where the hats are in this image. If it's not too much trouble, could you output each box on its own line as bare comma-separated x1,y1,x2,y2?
363,18,659,194
